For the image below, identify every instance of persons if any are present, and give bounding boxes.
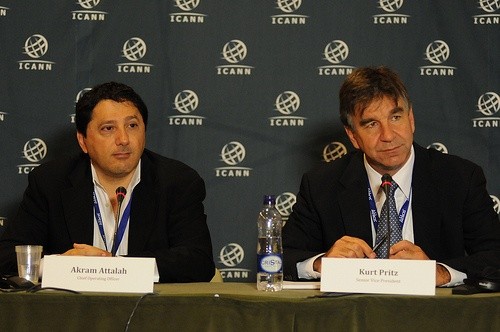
[0,81,217,285]
[279,66,499,288]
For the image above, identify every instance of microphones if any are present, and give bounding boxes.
[111,186,127,257]
[380,173,393,259]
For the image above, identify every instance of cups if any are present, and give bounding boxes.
[15,245,42,285]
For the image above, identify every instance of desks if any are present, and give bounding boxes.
[0,282,500,332]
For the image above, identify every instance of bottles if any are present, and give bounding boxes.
[255,194,285,293]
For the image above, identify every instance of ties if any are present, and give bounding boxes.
[374,176,402,259]
